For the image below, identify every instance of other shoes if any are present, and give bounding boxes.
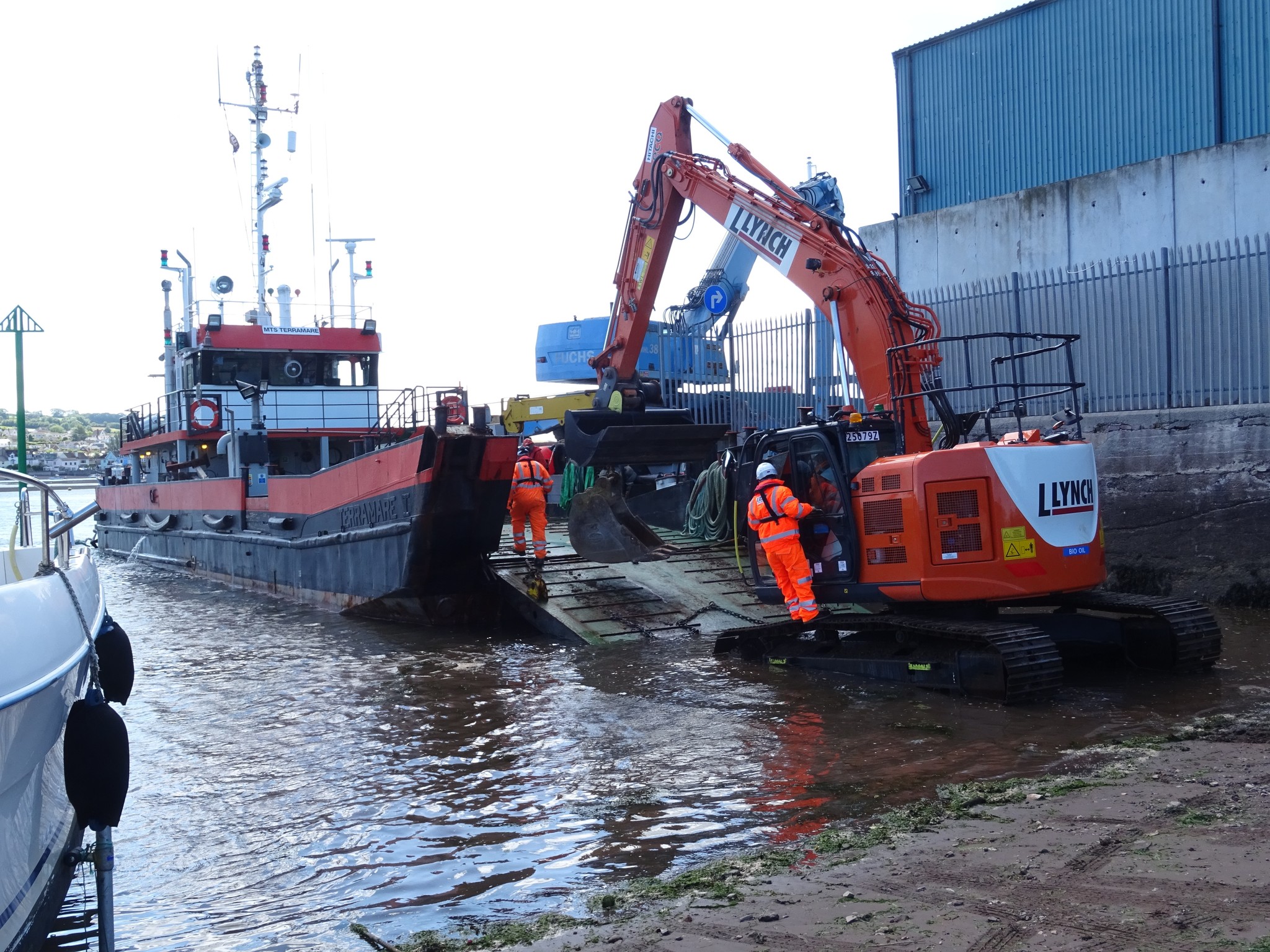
[803,610,834,624]
[513,544,526,556]
[792,618,803,623]
[534,556,544,566]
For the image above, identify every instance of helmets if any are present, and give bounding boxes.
[517,446,529,455]
[798,460,813,472]
[756,463,777,480]
[523,438,534,446]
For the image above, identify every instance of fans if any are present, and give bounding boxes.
[284,354,302,378]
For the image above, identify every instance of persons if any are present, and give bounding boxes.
[522,438,549,469]
[795,460,842,579]
[506,446,554,566]
[747,462,832,625]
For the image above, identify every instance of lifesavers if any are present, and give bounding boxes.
[441,395,466,425]
[190,399,219,430]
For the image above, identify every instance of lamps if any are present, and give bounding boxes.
[256,379,268,394]
[257,111,268,120]
[471,404,492,427]
[205,314,221,331]
[201,440,207,449]
[906,175,930,194]
[210,275,234,294]
[158,353,165,362]
[240,386,256,400]
[176,332,188,352]
[361,319,376,336]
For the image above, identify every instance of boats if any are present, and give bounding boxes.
[93,44,521,622]
[0,728,89,952]
[0,467,104,795]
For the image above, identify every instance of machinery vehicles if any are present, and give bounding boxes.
[500,94,1225,707]
